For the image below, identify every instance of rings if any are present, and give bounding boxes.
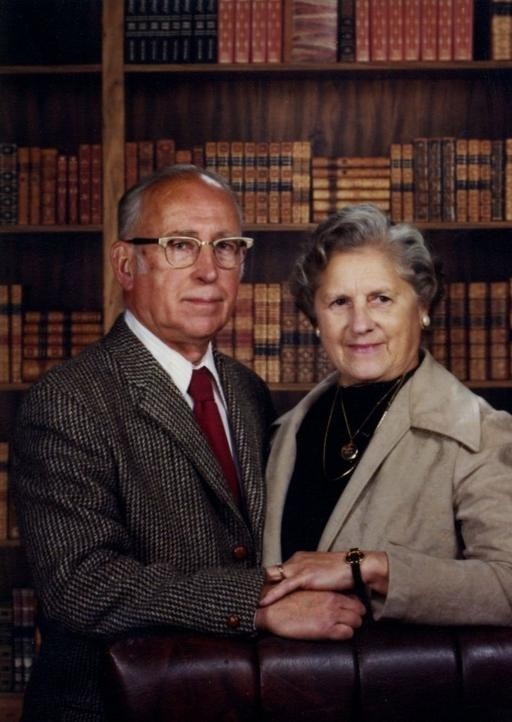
[278,564,286,581]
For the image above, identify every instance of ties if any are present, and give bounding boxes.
[187,367,242,517]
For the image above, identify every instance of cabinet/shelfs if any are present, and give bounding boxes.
[0,0,512,722]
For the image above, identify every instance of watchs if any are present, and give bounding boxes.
[344,547,365,590]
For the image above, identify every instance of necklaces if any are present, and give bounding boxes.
[319,379,407,483]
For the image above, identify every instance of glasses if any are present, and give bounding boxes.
[121,234,254,270]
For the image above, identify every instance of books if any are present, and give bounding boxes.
[126,138,511,223]
[0,3,510,65]
[423,283,511,380]
[0,284,103,383]
[1,145,105,224]
[0,443,41,692]
[213,284,336,385]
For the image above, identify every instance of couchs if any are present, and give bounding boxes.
[103,622,512,722]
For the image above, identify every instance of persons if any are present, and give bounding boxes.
[20,165,368,722]
[258,205,512,627]
